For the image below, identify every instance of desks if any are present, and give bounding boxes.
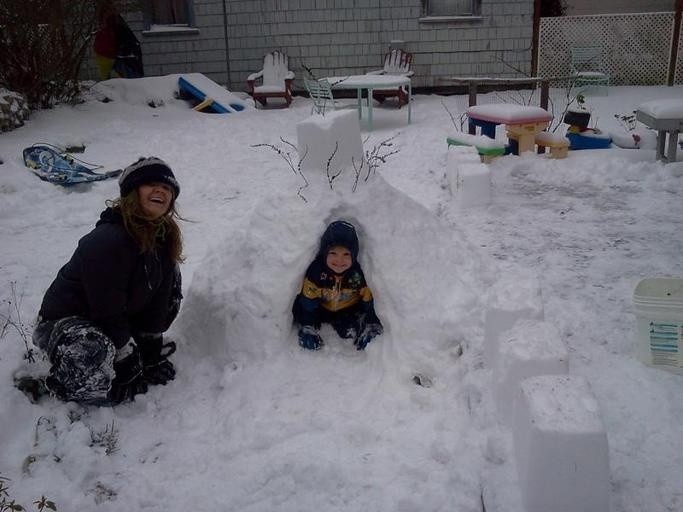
[465,106,553,156]
[318,75,412,136]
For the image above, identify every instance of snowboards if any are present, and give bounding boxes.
[23,146,123,186]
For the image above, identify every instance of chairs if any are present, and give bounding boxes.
[366,49,412,103]
[304,77,339,115]
[247,48,295,108]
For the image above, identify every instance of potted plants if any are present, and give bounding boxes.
[563,91,591,127]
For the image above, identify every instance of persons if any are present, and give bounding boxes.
[9,153,202,410]
[289,219,384,353]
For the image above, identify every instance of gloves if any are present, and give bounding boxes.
[354,323,384,351]
[297,322,323,350]
[110,348,148,406]
[137,334,175,386]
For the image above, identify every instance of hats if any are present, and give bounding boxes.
[319,220,360,266]
[119,156,180,199]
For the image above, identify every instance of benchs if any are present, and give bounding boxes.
[507,132,571,160]
[446,136,505,164]
[636,110,683,163]
